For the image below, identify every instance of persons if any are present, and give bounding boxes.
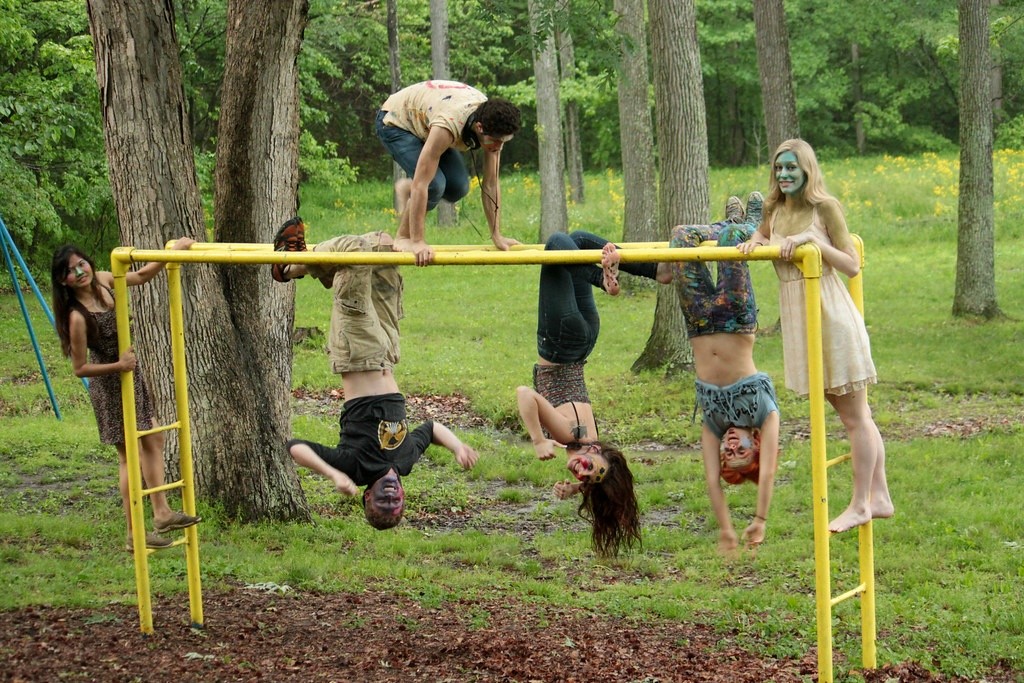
[517,231,672,560]
[375,79,521,267]
[737,140,894,533]
[271,217,481,531]
[669,192,779,559]
[52,237,202,548]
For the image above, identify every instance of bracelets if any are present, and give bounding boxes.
[755,514,766,520]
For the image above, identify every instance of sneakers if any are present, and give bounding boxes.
[271,219,298,282]
[292,216,309,279]
[726,196,746,224]
[153,510,202,534]
[746,191,764,223]
[126,530,173,551]
